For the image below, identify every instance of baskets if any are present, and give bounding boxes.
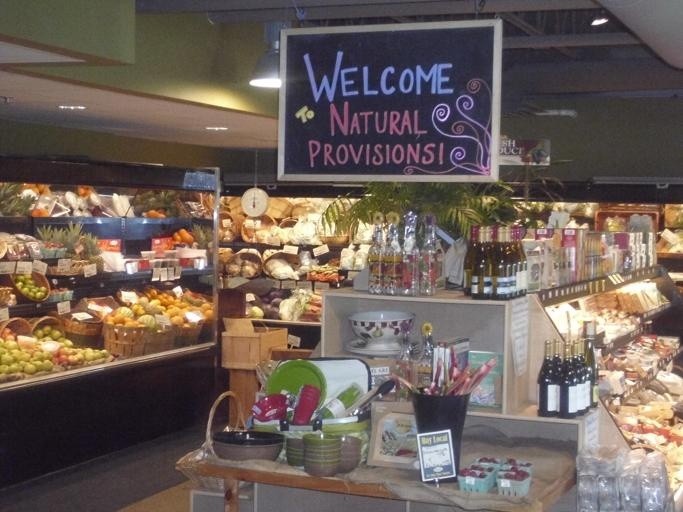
[176,194,350,290]
[175,391,252,491]
[1,257,213,358]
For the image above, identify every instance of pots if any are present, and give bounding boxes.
[286,433,362,477]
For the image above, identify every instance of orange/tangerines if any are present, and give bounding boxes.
[144,286,213,327]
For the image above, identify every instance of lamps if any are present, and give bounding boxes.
[587,8,609,27]
[249,19,292,89]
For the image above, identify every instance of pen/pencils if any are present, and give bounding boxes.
[391,342,498,398]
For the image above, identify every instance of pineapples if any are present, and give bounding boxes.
[36,222,105,275]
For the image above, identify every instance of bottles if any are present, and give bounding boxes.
[396,331,436,402]
[368,211,446,296]
[464,223,542,300]
[536,336,599,418]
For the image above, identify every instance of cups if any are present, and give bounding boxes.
[293,384,321,425]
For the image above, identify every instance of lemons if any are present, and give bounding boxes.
[106,315,145,330]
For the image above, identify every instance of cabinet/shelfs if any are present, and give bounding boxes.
[213,195,682,323]
[0,156,215,386]
[534,264,683,510]
[188,289,603,508]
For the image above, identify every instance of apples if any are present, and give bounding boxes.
[0,274,110,381]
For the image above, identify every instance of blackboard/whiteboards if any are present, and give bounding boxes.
[277,19,504,183]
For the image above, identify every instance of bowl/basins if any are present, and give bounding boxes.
[348,310,415,350]
[256,394,287,422]
[213,431,284,461]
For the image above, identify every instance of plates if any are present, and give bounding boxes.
[265,360,327,418]
[346,339,401,356]
